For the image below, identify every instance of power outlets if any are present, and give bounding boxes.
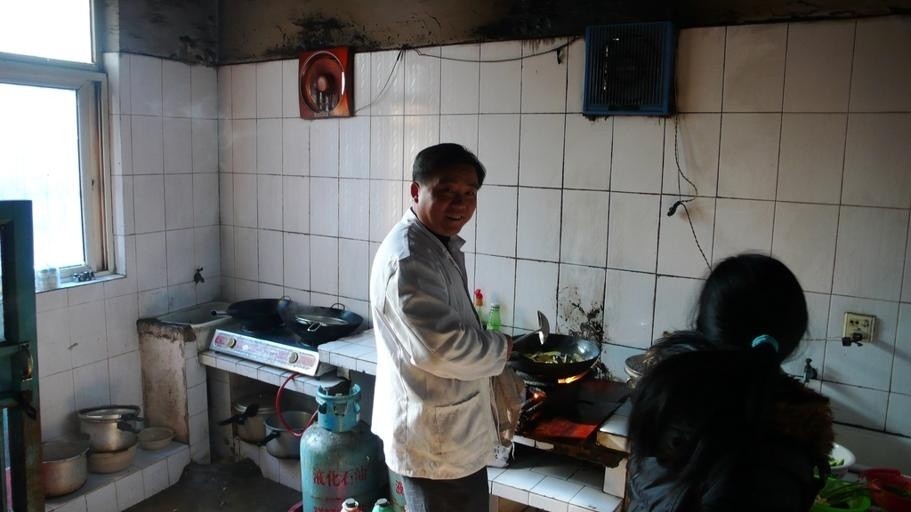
[842,311,876,344]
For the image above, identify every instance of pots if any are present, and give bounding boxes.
[41,440,89,499]
[504,333,601,379]
[76,401,144,453]
[219,390,289,444]
[257,411,316,459]
[211,293,294,329]
[284,301,364,344]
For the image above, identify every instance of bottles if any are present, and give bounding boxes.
[40,266,58,290]
[473,288,484,331]
[486,302,502,331]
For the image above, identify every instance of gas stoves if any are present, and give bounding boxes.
[208,324,335,378]
[514,369,594,433]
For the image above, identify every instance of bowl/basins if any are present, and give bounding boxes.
[828,442,857,478]
[89,440,140,475]
[137,426,175,451]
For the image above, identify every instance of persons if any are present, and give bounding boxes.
[623,254,834,511]
[370,143,512,512]
